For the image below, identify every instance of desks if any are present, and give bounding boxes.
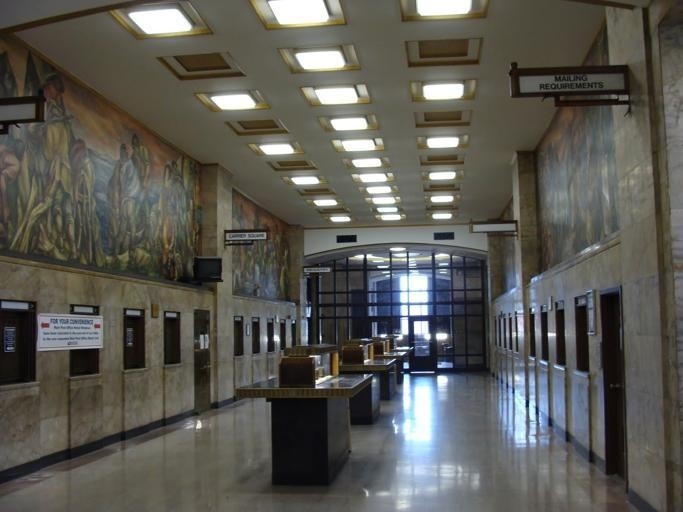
[235,347,415,485]
